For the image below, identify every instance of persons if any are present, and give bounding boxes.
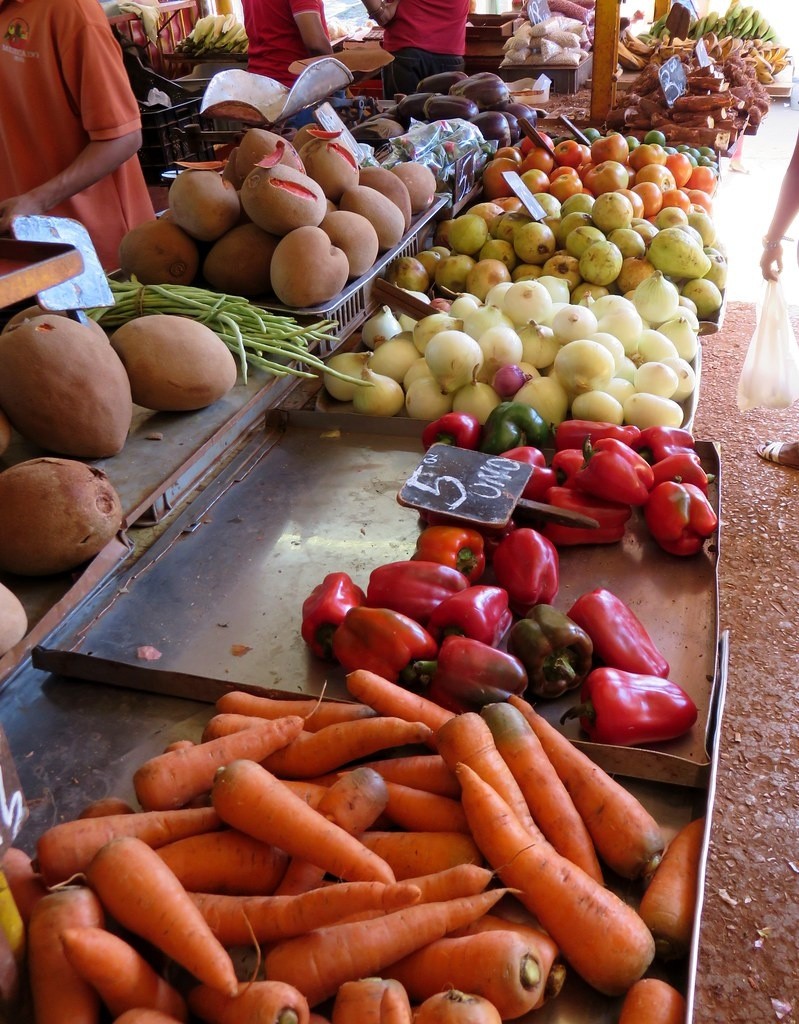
[239,0,334,91]
[755,129,799,470]
[1,0,161,277]
[361,0,477,101]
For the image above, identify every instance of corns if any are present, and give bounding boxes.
[175,13,249,54]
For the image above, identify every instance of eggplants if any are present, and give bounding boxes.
[347,71,537,147]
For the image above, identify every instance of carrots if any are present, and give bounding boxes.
[1,667,705,1024]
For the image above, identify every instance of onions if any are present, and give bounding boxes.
[322,277,699,432]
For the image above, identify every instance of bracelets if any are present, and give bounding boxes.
[367,2,386,20]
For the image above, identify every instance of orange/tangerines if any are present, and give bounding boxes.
[553,127,718,170]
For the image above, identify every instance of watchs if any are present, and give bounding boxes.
[762,235,781,251]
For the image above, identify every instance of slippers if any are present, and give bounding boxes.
[758,441,799,471]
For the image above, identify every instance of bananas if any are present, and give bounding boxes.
[615,0,791,84]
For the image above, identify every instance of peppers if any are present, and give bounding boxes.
[298,402,717,745]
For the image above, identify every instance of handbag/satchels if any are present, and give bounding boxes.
[737,270,799,412]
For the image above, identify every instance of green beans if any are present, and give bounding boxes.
[83,274,373,387]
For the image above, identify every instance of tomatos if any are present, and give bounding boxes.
[468,132,717,225]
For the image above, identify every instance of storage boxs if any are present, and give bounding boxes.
[497,51,594,96]
[137,96,219,188]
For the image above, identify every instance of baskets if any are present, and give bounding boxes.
[242,196,451,359]
[499,51,593,94]
